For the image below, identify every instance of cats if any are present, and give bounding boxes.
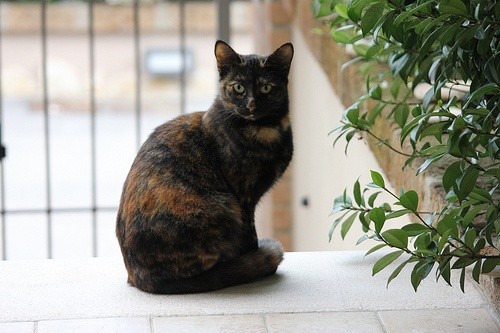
[115,40,294,295]
